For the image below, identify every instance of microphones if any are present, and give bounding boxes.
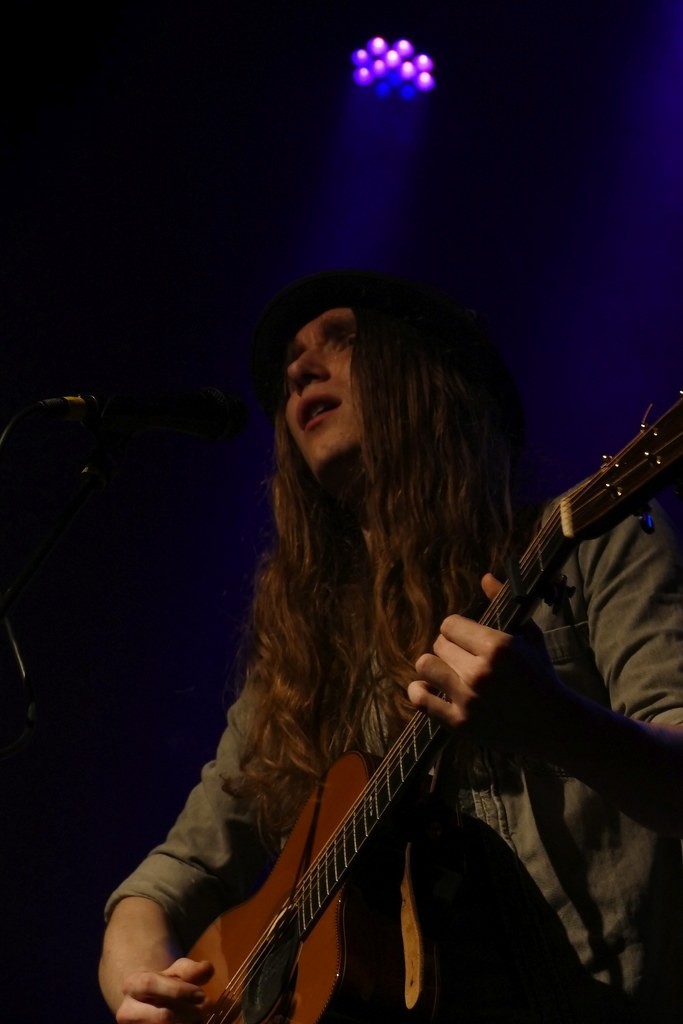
[39,386,250,442]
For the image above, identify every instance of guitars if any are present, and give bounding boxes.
[177,380,673,1023]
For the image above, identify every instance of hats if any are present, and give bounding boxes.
[252,270,527,450]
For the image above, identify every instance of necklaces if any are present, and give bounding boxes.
[362,571,387,750]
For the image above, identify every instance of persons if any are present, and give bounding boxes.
[99,269,683,1024]
